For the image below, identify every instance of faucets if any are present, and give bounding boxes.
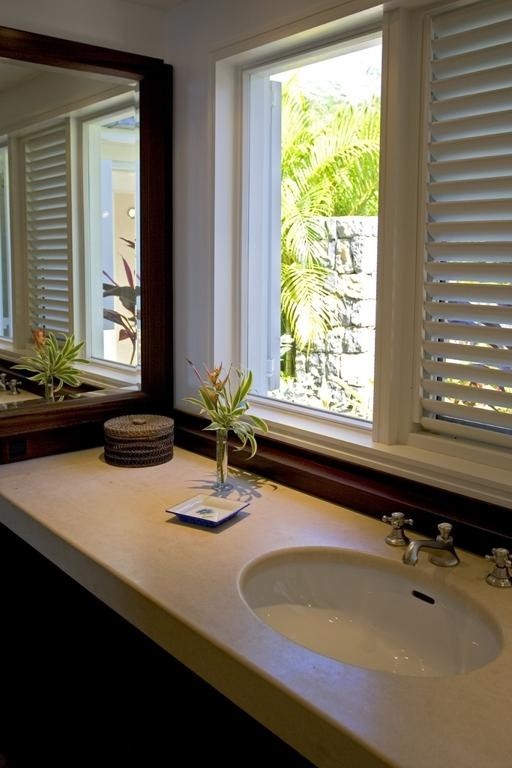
[401,521,461,568]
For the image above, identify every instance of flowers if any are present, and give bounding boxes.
[10,326,90,403]
[181,357,268,461]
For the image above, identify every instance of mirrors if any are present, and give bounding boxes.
[0,26,173,464]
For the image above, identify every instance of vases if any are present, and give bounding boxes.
[45,377,54,402]
[212,428,233,492]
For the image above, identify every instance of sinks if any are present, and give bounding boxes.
[235,545,501,677]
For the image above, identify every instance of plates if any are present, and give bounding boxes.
[166,494,250,528]
